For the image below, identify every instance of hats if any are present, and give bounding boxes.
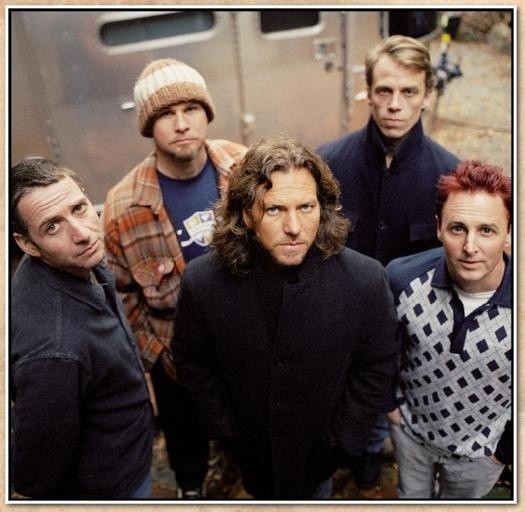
[132,59,214,138]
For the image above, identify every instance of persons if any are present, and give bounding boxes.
[383,156,515,501]
[9,154,156,498]
[314,33,473,502]
[101,55,253,500]
[167,132,406,501]
